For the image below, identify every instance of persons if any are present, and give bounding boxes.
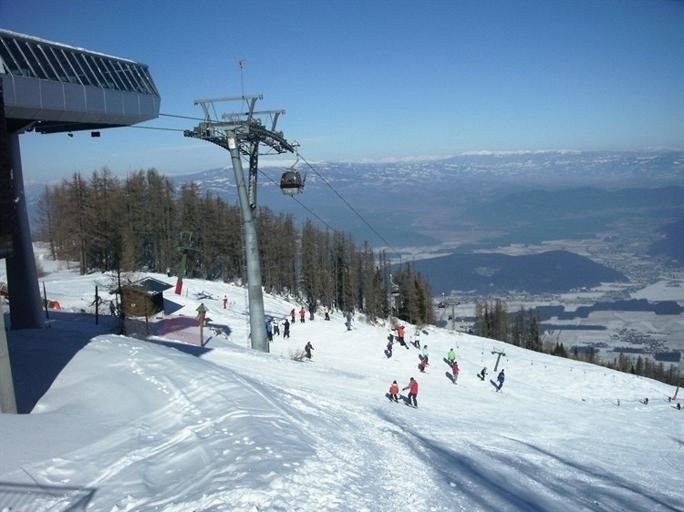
[481,367,487,380]
[345,310,353,330]
[403,377,418,408]
[305,342,314,359]
[265,302,330,341]
[447,349,455,365]
[389,380,399,401]
[452,361,459,384]
[110,301,116,316]
[385,325,429,372]
[497,369,504,389]
[222,295,228,309]
